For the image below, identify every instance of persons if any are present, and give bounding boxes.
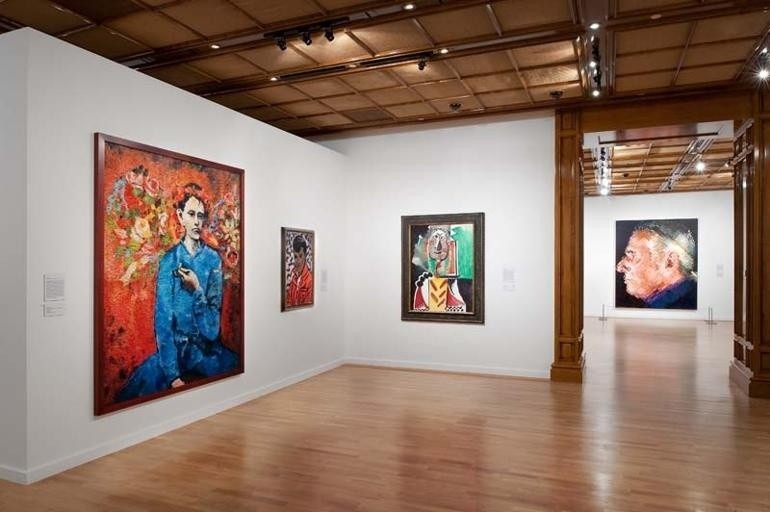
[286,235,313,306]
[115,182,239,403]
[616,220,698,310]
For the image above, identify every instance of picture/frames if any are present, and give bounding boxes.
[88,129,248,421]
[398,211,487,326]
[277,223,317,315]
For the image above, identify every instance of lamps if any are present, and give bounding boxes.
[416,54,427,71]
[590,31,605,96]
[257,11,353,54]
